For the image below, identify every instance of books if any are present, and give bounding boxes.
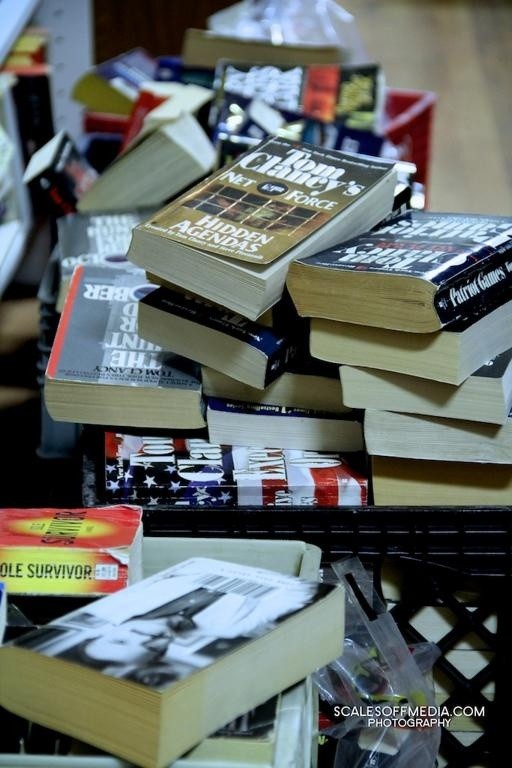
[205,59,387,157]
[136,286,310,391]
[72,46,162,116]
[287,212,512,333]
[200,356,353,413]
[77,115,219,214]
[125,138,399,322]
[339,353,512,427]
[0,702,282,768]
[23,129,98,216]
[307,300,512,385]
[370,452,512,509]
[42,266,207,428]
[205,394,363,451]
[52,208,158,314]
[105,431,370,512]
[0,506,146,597]
[363,405,512,466]
